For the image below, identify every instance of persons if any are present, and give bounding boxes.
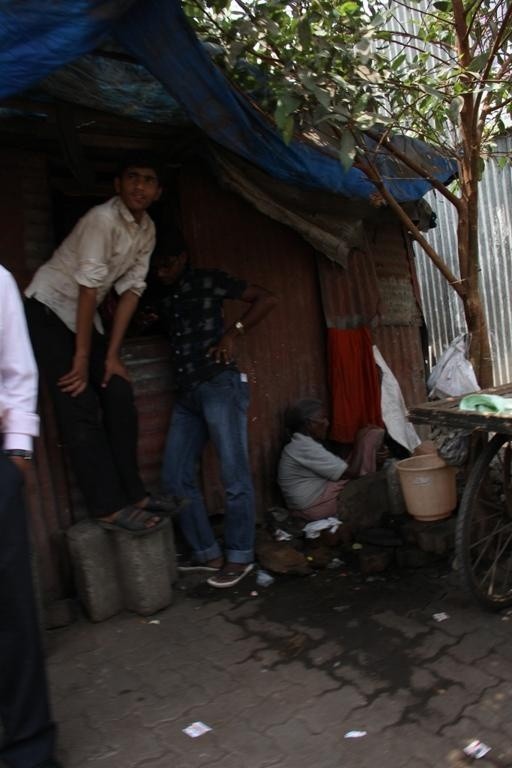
[22,150,170,535]
[1,263,63,768]
[276,397,391,528]
[130,226,282,589]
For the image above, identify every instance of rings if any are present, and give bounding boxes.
[222,348,227,352]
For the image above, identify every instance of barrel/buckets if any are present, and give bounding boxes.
[394,454,461,523]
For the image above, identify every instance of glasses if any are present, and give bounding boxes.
[153,258,180,271]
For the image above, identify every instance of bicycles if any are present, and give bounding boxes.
[410,380,512,614]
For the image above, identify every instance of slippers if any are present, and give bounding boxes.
[207,560,258,588]
[137,493,187,516]
[175,557,225,573]
[100,507,170,536]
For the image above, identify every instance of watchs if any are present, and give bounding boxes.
[4,449,32,462]
[230,320,245,335]
